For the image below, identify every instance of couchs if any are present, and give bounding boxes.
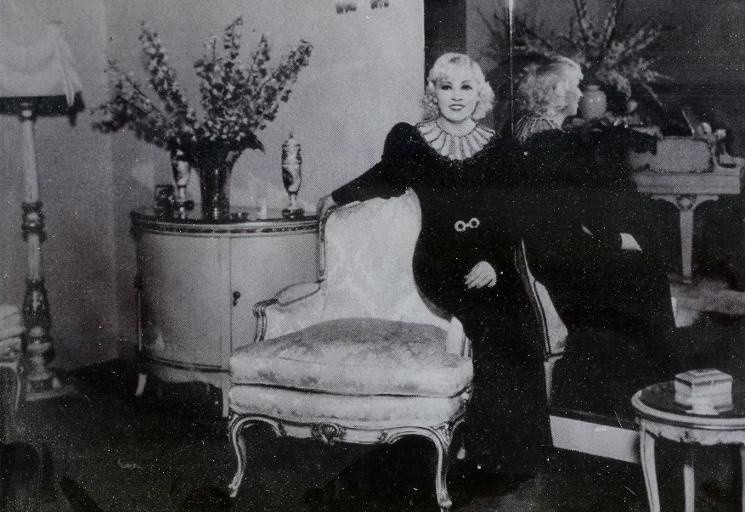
[228,190,475,508]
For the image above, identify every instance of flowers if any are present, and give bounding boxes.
[91,18,315,165]
[507,0,678,114]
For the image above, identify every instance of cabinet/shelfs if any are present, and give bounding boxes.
[130,205,320,418]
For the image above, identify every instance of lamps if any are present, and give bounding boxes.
[0,20,84,401]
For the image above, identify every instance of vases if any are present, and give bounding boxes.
[200,153,232,224]
[578,84,609,122]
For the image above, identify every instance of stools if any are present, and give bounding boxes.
[632,381,744,512]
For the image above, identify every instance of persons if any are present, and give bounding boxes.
[317,51,554,496]
[500,54,679,423]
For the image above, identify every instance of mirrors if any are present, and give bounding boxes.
[423,1,744,431]
